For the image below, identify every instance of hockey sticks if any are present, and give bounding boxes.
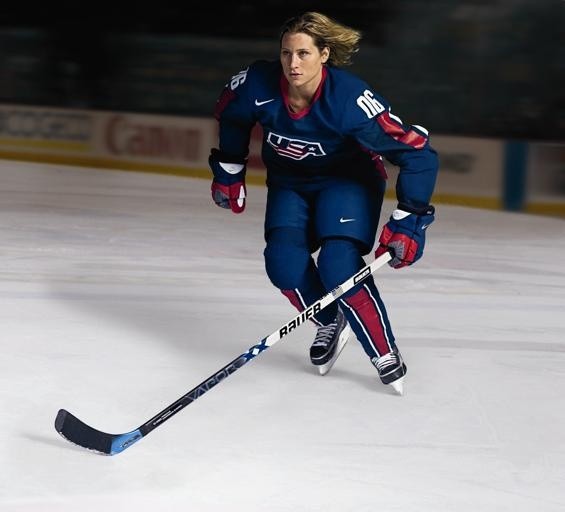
[55,205,437,454]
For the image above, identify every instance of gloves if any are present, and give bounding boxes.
[208,147,246,212]
[373,203,434,270]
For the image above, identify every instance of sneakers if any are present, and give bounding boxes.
[370,345,408,384]
[310,312,344,366]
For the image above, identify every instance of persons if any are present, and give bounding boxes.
[208,11,439,385]
